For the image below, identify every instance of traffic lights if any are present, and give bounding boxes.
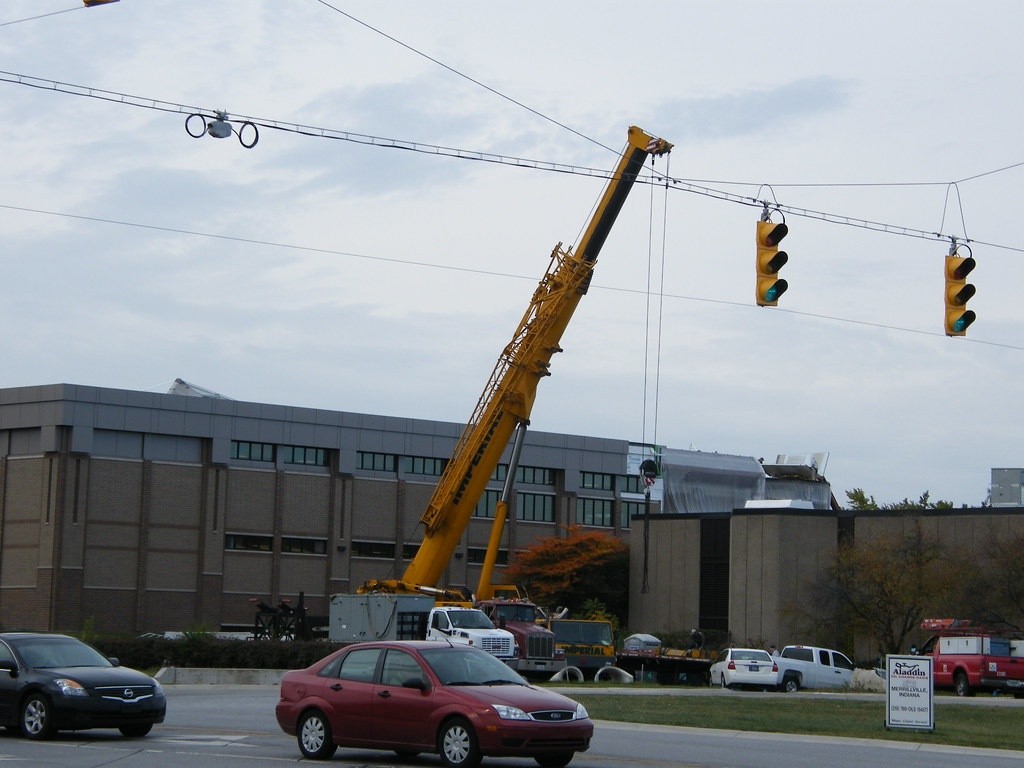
[756,219,789,308]
[943,255,978,335]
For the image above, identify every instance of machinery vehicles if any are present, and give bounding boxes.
[355,125,676,667]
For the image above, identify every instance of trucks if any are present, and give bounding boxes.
[329,593,568,679]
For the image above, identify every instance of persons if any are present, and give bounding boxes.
[770,645,780,657]
[691,629,705,649]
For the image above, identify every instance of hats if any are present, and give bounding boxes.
[691,629,696,635]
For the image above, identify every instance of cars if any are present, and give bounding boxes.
[709,648,778,691]
[0,632,167,739]
[276,640,594,768]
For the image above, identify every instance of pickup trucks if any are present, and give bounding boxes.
[771,645,860,693]
[909,618,1024,699]
